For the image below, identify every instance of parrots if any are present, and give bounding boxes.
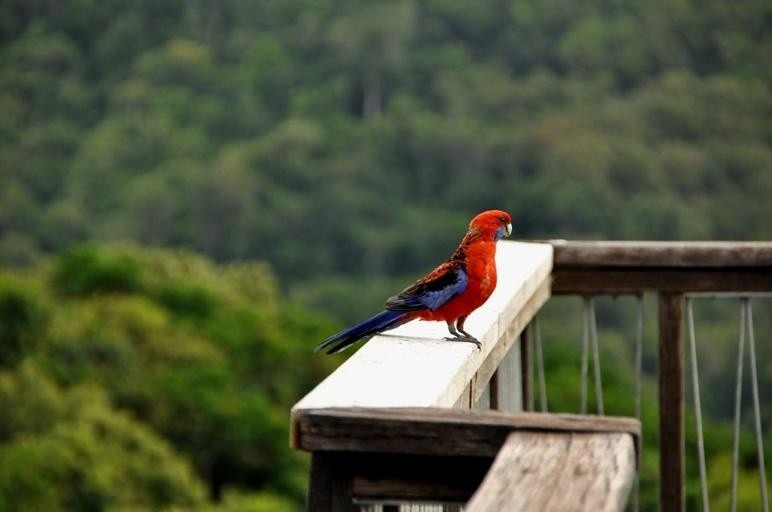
[314,208,514,356]
